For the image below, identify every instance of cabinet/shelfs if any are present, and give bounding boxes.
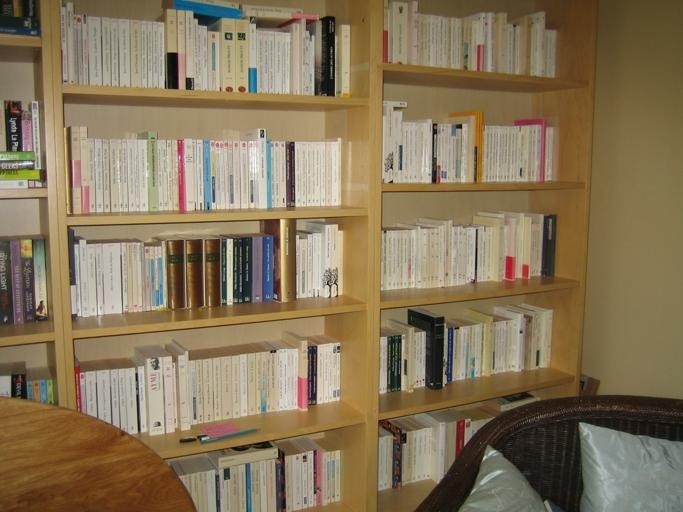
[367,0,601,512]
[53,1,374,512]
[0,1,68,408]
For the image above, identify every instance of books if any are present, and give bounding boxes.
[60,0,352,99]
[1,1,40,37]
[73,331,340,435]
[65,124,341,215]
[0,235,50,326]
[380,302,553,396]
[1,361,56,407]
[382,99,559,186]
[382,209,556,289]
[377,393,542,491]
[2,100,45,188]
[382,0,557,77]
[68,219,345,318]
[166,438,341,510]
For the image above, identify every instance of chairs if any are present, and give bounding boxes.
[413,394,683,512]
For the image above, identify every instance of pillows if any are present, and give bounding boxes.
[459,443,552,511]
[577,420,683,512]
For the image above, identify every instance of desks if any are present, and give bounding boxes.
[0,395,199,512]
